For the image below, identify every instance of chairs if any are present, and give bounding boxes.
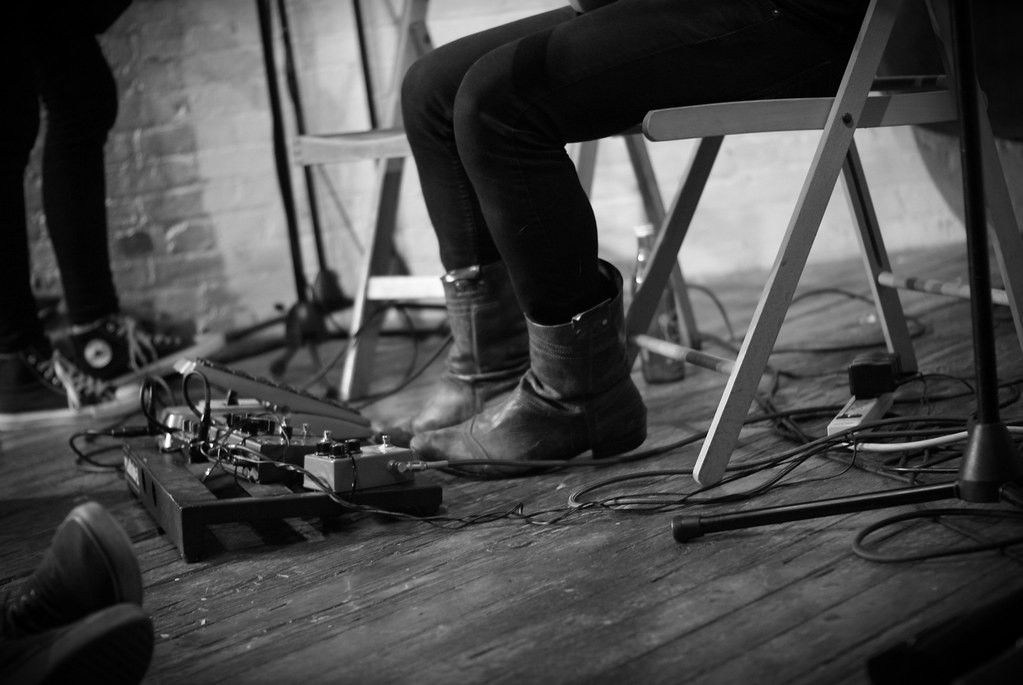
[296,0,1023,489]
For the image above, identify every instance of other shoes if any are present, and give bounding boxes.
[13,602,155,685]
[0,502,144,630]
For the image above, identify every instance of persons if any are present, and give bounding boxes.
[0,0,228,436]
[359,0,1023,480]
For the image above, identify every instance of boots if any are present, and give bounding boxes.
[370,263,528,446]
[412,258,648,480]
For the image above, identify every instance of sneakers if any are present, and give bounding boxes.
[68,316,225,386]
[0,339,150,431]
